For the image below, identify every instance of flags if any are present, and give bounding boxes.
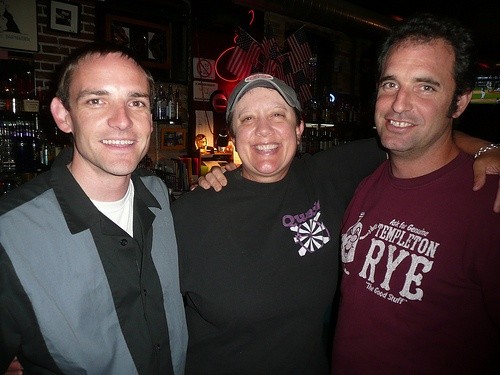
[222,16,318,105]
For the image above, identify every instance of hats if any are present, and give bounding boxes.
[226,71,302,118]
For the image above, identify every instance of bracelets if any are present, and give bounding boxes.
[473,143,499,160]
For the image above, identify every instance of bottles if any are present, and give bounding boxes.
[0,85,360,197]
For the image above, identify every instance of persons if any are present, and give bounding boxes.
[190,15,500,375]
[4,72,500,375]
[0,49,197,374]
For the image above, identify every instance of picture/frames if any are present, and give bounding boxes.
[0,0,39,53]
[47,1,82,36]
[160,128,186,150]
